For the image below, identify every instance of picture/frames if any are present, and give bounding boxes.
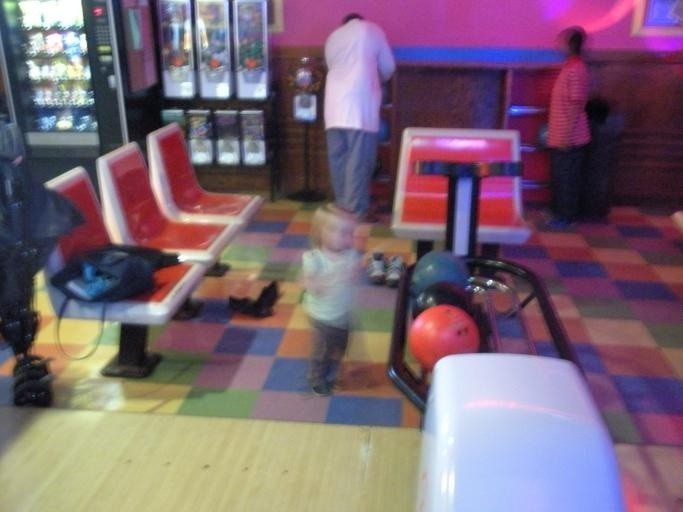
[629,1,683,38]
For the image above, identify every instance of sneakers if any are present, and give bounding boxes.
[310,381,329,397]
[366,251,386,282]
[386,255,403,286]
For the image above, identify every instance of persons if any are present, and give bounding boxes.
[322,14,396,220]
[547,27,592,232]
[298,204,368,397]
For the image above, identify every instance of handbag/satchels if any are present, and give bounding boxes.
[52,249,153,361]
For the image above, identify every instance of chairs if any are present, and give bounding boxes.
[146,123,262,276]
[39,164,206,379]
[390,127,531,288]
[94,139,240,322]
